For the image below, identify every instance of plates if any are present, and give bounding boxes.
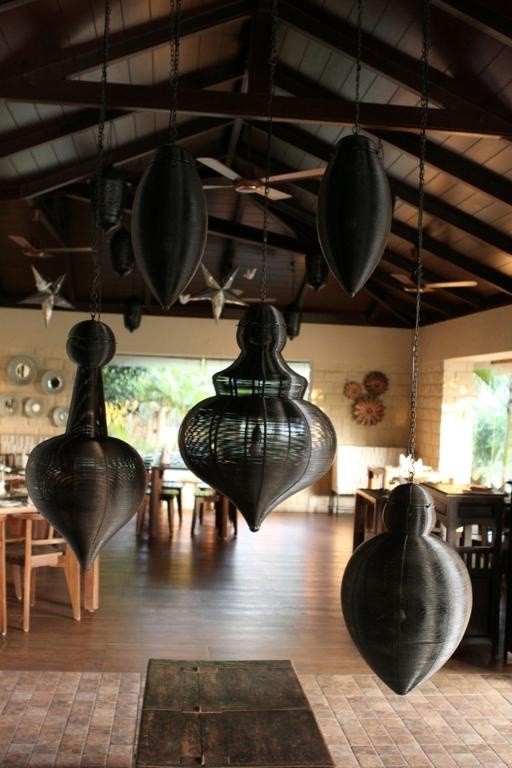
[0,354,69,427]
[470,486,493,491]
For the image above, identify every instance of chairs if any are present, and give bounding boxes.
[6,513,102,633]
